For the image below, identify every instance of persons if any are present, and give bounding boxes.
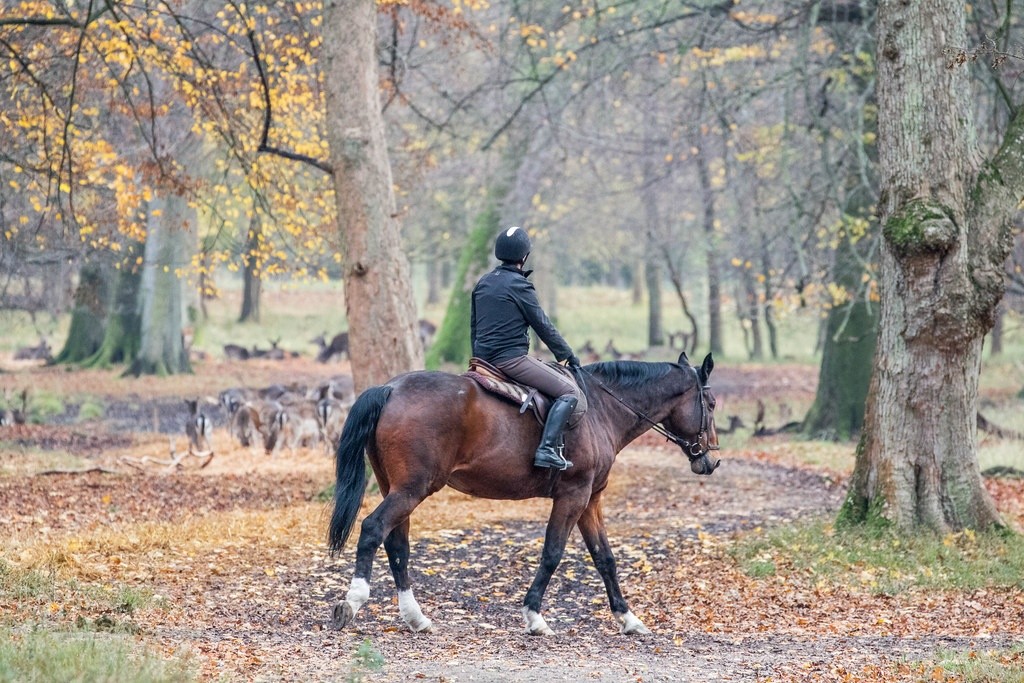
[471,225,581,468]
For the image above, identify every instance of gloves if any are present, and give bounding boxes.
[567,355,580,368]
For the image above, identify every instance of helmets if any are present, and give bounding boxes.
[494,227,530,262]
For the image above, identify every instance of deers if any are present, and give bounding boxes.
[182,321,766,457]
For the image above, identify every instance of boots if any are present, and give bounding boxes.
[534,395,573,468]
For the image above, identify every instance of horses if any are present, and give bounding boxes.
[322,346,727,635]
